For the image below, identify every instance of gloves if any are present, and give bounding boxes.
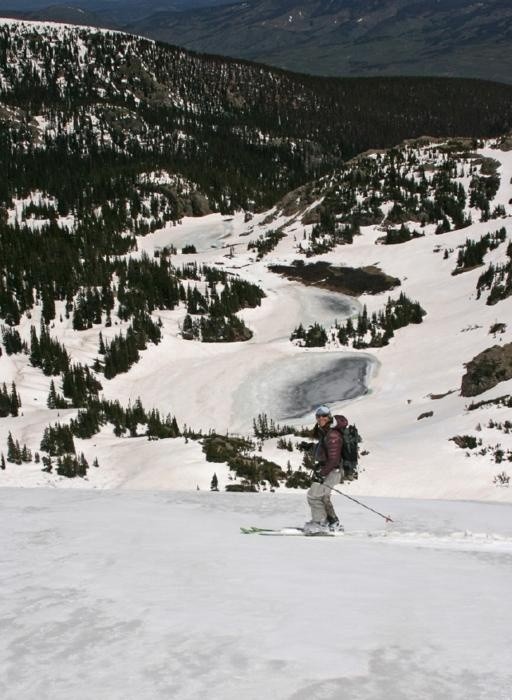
[313,470,324,485]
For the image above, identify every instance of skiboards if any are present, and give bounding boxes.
[240,527,388,539]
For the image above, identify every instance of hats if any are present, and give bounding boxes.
[316,406,331,418]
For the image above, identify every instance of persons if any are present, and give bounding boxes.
[303,405,343,533]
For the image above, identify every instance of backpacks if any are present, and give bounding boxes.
[323,423,358,481]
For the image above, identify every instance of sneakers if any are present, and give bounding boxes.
[328,520,340,529]
[305,519,326,529]
